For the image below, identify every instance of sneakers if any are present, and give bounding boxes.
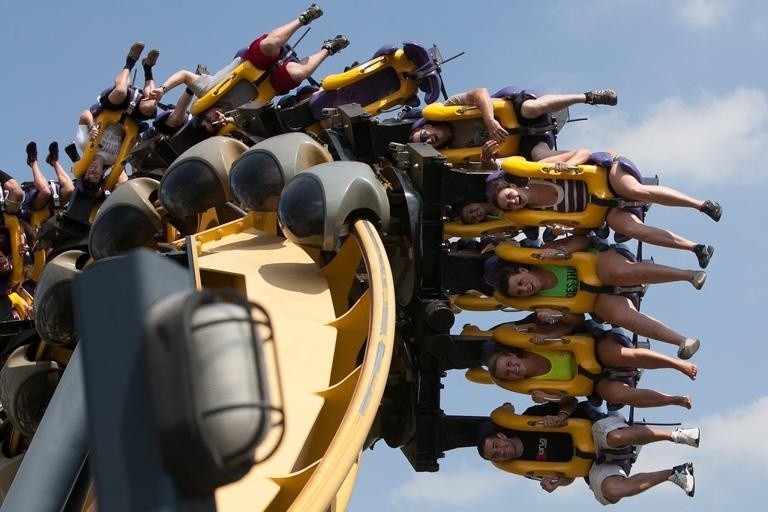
[677,337,700,360]
[195,63,209,76]
[127,41,145,62]
[693,244,714,268]
[45,141,59,166]
[583,88,618,105]
[667,460,696,497]
[690,270,707,290]
[698,198,723,222]
[321,34,351,57]
[671,426,701,448]
[141,49,160,68]
[25,141,38,167]
[298,3,323,26]
[64,141,80,163]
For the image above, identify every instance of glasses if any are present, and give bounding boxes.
[419,128,430,143]
